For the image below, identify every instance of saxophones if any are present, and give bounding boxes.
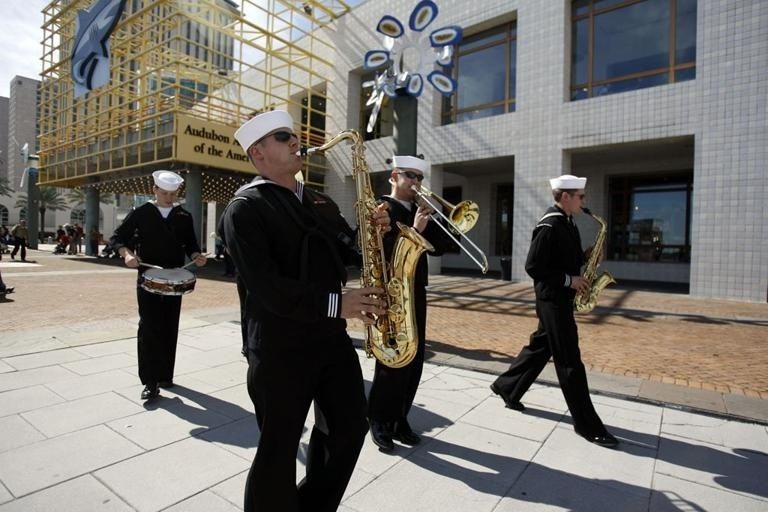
[295,128,435,369]
[574,206,616,315]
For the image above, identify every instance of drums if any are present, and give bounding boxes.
[140,267,197,296]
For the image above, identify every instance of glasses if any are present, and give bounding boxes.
[396,171,424,181]
[254,131,297,146]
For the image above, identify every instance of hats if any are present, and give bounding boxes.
[152,170,184,191]
[549,175,587,189]
[234,110,293,151]
[393,155,425,172]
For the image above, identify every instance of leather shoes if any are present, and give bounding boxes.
[490,382,525,411]
[141,382,160,399]
[576,430,619,447]
[393,432,420,445]
[367,417,395,449]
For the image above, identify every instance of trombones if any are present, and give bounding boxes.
[389,173,490,273]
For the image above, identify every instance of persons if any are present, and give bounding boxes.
[110,170,207,399]
[215,109,388,512]
[489,174,620,447]
[54,223,83,254]
[366,156,447,450]
[103,226,140,259]
[0,220,29,303]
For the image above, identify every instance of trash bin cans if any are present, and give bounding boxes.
[80,236,84,244]
[47,235,53,245]
[498,257,512,280]
